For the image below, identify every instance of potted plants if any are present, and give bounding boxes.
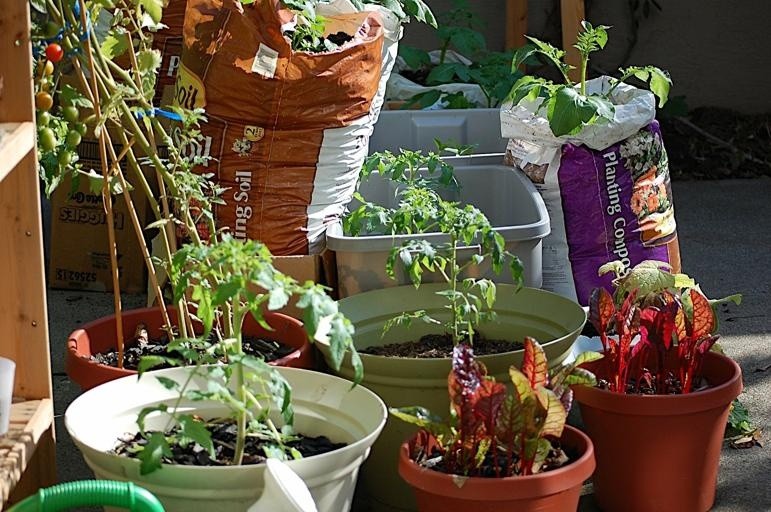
[569,260,744,512]
[65,235,387,512]
[326,165,550,300]
[368,28,541,168]
[29,0,308,394]
[314,187,588,512]
[388,336,604,512]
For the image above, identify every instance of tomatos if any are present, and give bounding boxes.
[32,42,87,166]
